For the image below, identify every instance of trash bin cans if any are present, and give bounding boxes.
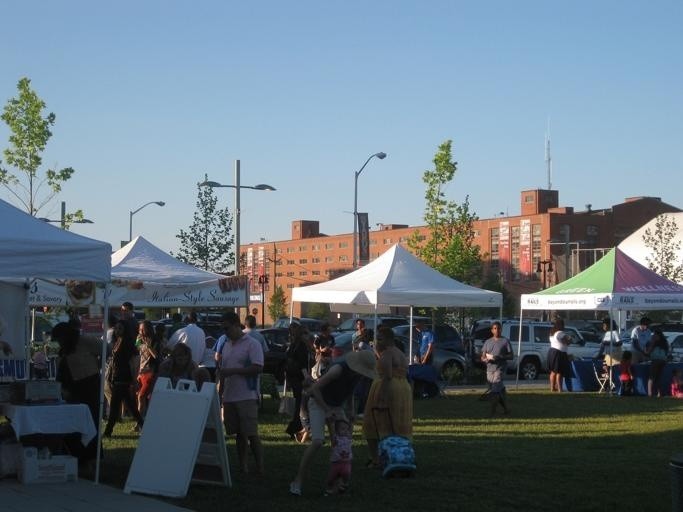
[670,453,683,512]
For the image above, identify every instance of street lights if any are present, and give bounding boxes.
[257,274,269,328]
[353,151,388,270]
[199,158,278,321]
[127,201,166,242]
[30,202,94,232]
[535,260,553,322]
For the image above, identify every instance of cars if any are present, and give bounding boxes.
[255,328,294,366]
[29,311,72,344]
[326,316,468,384]
[77,310,246,362]
[574,323,682,364]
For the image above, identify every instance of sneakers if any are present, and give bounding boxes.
[289,480,301,495]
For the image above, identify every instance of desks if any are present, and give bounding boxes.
[0,399,99,460]
[605,359,682,399]
[407,362,437,397]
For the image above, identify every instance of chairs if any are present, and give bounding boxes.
[436,361,459,398]
[591,358,616,394]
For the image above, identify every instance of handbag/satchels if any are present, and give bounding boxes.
[278,397,295,417]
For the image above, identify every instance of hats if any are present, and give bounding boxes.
[345,350,377,381]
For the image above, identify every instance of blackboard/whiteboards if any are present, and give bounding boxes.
[125,377,216,499]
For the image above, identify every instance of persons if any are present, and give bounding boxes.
[51,301,413,499]
[548,319,572,392]
[630,317,652,363]
[593,319,622,395]
[619,351,634,396]
[481,322,514,418]
[670,369,683,399]
[415,322,441,399]
[644,329,669,398]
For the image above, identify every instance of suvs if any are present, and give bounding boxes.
[270,316,330,344]
[468,319,604,380]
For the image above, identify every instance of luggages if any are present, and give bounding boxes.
[370,407,417,479]
